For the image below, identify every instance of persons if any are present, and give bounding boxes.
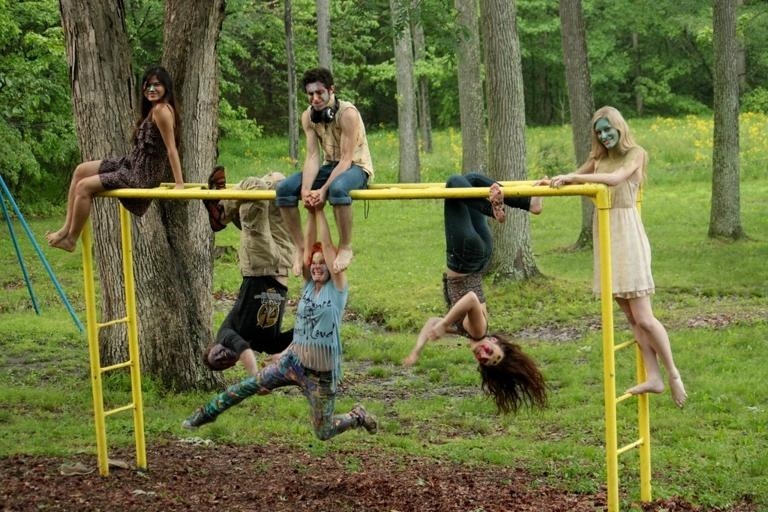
[551,106,688,409]
[182,192,378,441]
[404,171,550,418]
[44,67,183,251]
[200,166,295,395]
[275,67,374,277]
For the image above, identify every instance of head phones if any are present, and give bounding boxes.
[311,106,335,124]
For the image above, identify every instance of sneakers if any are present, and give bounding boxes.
[183,409,217,429]
[202,168,227,231]
[353,404,377,436]
